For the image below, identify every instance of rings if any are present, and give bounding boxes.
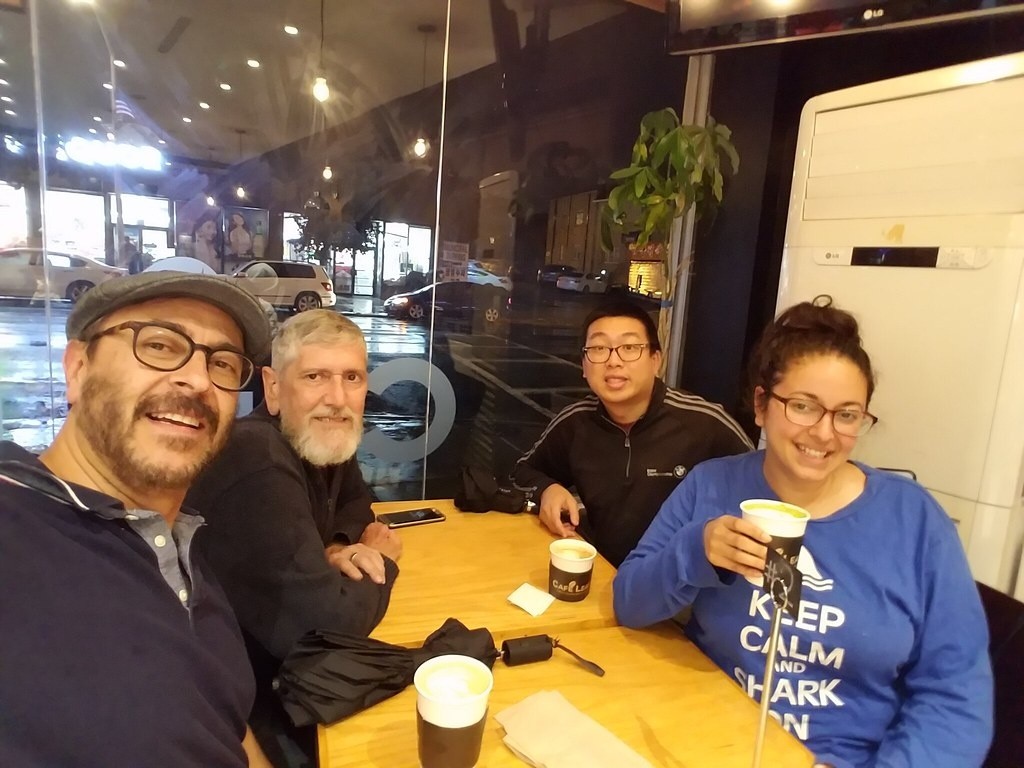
[350,552,358,562]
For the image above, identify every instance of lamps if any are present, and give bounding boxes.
[236,185,245,197]
[323,162,333,178]
[313,65,329,102]
[415,130,428,159]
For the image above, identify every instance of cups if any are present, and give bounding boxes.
[414,654,493,768]
[739,498,811,588]
[548,538,597,603]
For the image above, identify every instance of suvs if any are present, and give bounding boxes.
[217,259,337,317]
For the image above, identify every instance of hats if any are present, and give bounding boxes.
[66,256,270,369]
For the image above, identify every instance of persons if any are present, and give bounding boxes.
[227,212,252,257]
[124,236,136,260]
[130,252,144,276]
[0,268,277,768]
[179,310,403,767]
[610,295,998,768]
[191,212,221,273]
[494,301,754,571]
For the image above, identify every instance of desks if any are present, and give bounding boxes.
[318,499,826,768]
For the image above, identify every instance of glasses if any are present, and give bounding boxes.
[768,391,878,437]
[88,322,259,391]
[582,344,650,364]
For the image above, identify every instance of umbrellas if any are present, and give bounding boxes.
[272,616,604,729]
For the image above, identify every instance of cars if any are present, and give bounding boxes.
[536,264,573,285]
[467,261,514,293]
[382,282,513,322]
[0,248,130,304]
[556,269,612,296]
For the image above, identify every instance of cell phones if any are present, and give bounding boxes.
[376,508,446,529]
